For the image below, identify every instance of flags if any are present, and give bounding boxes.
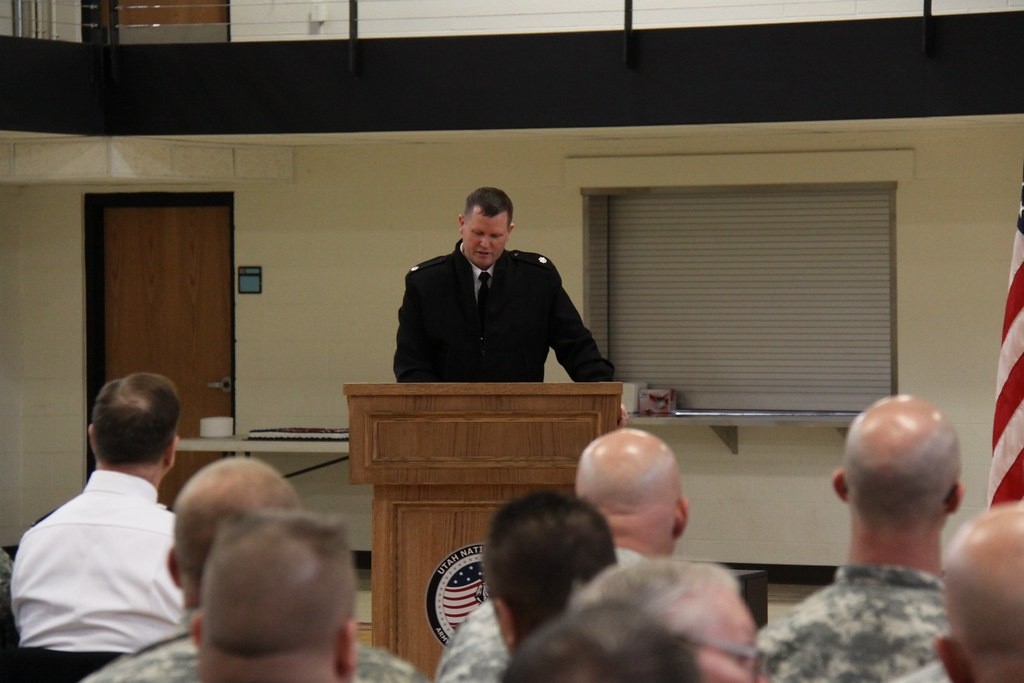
[987,169,1024,507]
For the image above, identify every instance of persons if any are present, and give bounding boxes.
[498,596,705,683]
[434,428,688,683]
[935,501,1024,683]
[482,491,620,663]
[78,456,428,683]
[192,503,359,683]
[392,186,629,430]
[10,372,190,655]
[567,556,770,683]
[755,394,966,683]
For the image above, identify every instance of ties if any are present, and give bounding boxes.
[478,272,491,310]
